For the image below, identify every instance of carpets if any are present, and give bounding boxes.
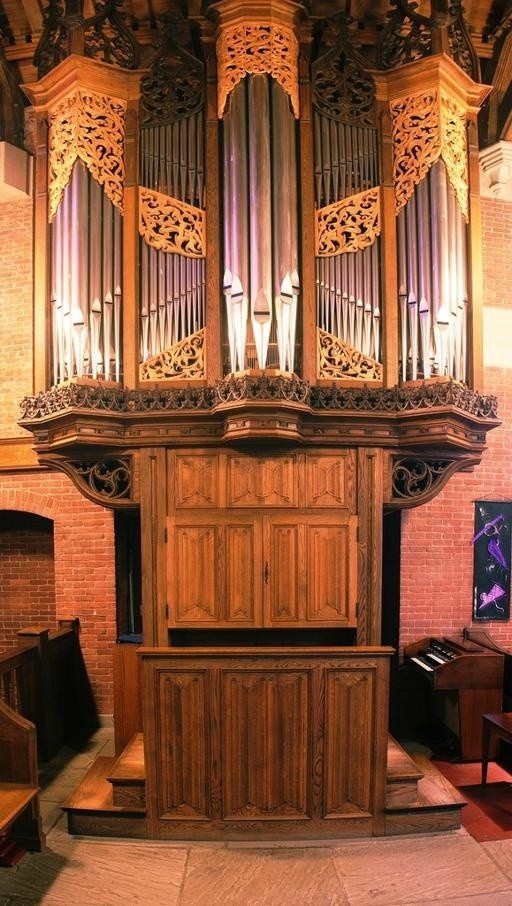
[449,780,511,842]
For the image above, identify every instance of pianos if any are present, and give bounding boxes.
[402,636,505,761]
